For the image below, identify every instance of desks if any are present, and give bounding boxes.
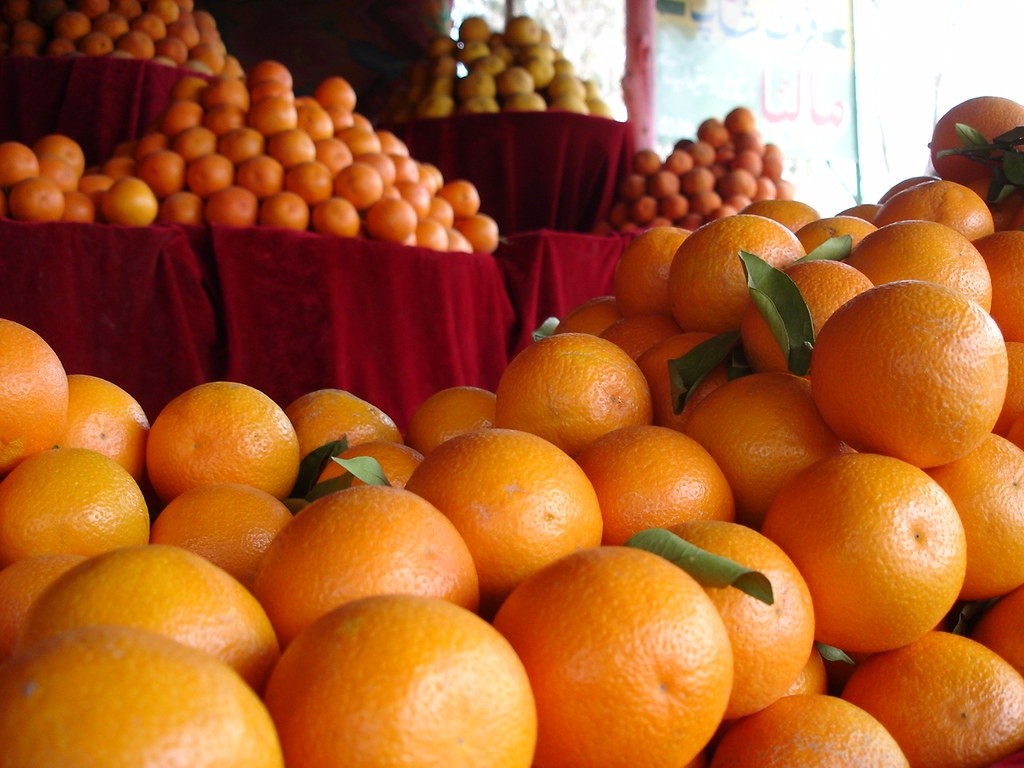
[0,0,651,408]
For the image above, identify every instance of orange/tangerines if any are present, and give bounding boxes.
[1,94,1024,768]
[0,0,500,256]
[380,15,609,119]
